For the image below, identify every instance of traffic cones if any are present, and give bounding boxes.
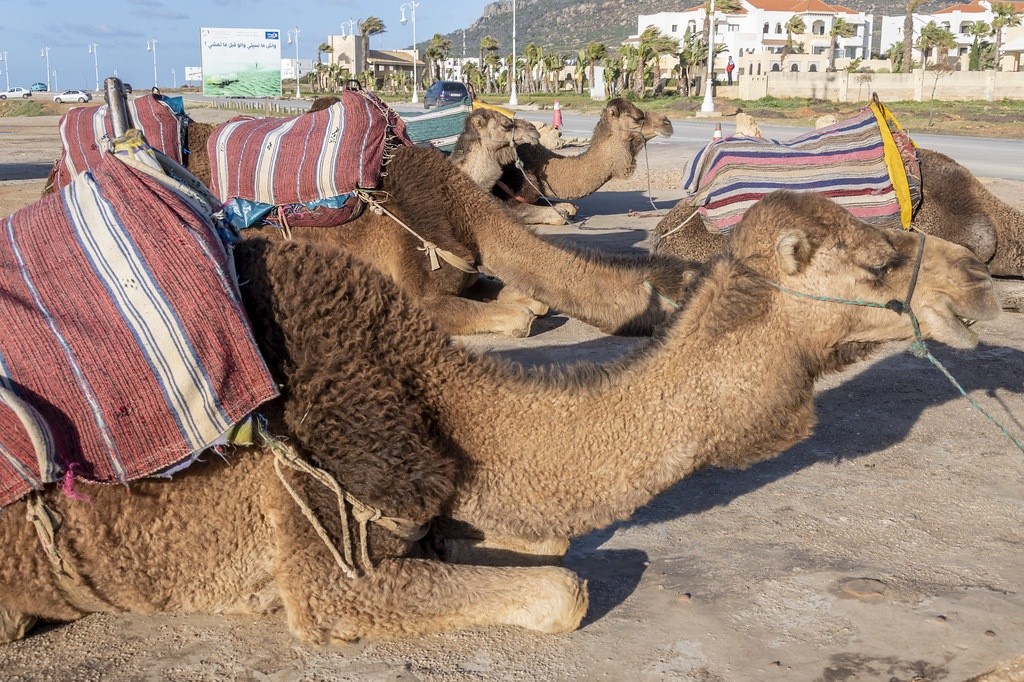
[551,100,563,128]
[713,123,722,141]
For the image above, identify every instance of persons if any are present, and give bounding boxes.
[726,55,735,85]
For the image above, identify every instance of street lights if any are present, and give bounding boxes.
[88,42,100,92]
[341,17,357,87]
[41,45,51,91]
[114,69,117,78]
[53,70,57,92]
[400,0,419,103]
[147,38,158,88]
[172,68,176,89]
[287,27,302,99]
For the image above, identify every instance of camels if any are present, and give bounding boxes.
[0,73,1024,645]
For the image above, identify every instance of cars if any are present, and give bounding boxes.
[31,83,47,92]
[54,90,92,103]
[0,87,32,100]
[123,83,132,94]
[0,49,9,93]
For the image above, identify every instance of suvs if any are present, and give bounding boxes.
[424,81,468,109]
